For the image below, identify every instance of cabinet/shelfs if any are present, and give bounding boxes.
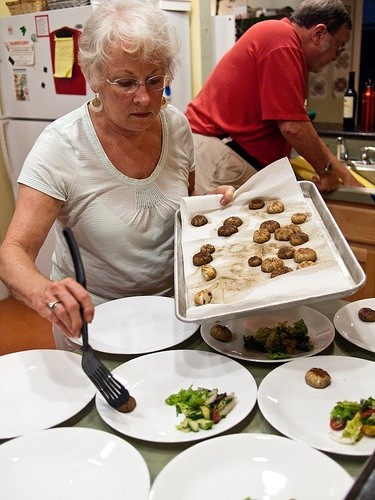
[326,200,375,302]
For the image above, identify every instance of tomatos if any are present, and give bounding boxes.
[330,417,346,430]
[210,409,219,424]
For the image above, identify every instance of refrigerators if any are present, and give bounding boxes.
[1,6,193,284]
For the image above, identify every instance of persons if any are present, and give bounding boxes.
[0,1,236,349]
[184,0,356,193]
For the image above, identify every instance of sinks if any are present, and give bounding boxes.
[331,162,375,192]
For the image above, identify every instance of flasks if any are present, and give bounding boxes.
[357,77,375,133]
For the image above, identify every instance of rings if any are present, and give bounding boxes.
[48,300,61,311]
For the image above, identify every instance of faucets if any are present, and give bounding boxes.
[336,136,349,160]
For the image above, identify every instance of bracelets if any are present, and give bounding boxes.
[320,159,337,175]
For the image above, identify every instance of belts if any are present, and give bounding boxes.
[223,137,264,172]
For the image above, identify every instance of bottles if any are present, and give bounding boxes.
[336,137,348,162]
[342,70,357,131]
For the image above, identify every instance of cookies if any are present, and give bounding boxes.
[210,323,232,342]
[189,199,318,305]
[305,368,332,389]
[116,396,136,413]
[357,308,375,322]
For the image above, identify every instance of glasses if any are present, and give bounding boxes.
[102,67,175,94]
[332,34,346,57]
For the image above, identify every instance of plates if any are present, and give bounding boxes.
[0,427,151,500]
[95,348,258,444]
[0,348,98,441]
[257,355,375,456]
[198,304,336,363]
[148,431,358,500]
[68,295,202,354]
[332,297,375,355]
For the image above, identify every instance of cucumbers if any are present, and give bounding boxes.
[197,418,213,430]
[188,421,199,432]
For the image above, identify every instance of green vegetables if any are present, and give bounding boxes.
[330,396,375,444]
[241,318,313,360]
[165,384,227,432]
